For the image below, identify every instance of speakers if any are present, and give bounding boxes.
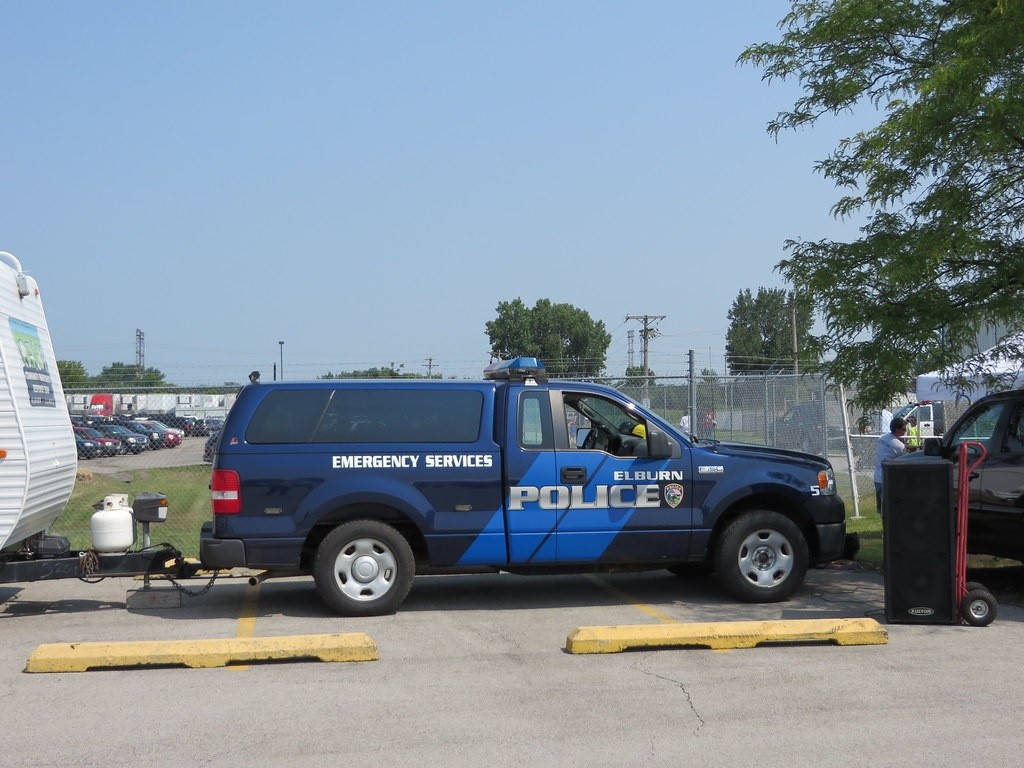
[883,450,954,625]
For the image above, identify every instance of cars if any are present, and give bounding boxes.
[75,433,100,460]
[70,414,133,426]
[150,414,226,437]
[895,388,1024,564]
[145,420,185,448]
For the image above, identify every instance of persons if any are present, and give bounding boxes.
[679,408,717,439]
[879,406,894,434]
[568,413,579,447]
[874,416,918,519]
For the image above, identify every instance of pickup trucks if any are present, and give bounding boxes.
[201,370,860,618]
[765,400,857,456]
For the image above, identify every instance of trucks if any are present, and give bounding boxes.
[896,400,996,439]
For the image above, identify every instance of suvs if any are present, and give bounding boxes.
[73,427,121,457]
[121,421,166,450]
[99,425,150,455]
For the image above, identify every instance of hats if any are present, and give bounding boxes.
[910,417,917,425]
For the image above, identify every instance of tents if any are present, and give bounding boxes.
[916,331,1024,453]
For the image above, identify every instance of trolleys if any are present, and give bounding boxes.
[954,441,998,627]
